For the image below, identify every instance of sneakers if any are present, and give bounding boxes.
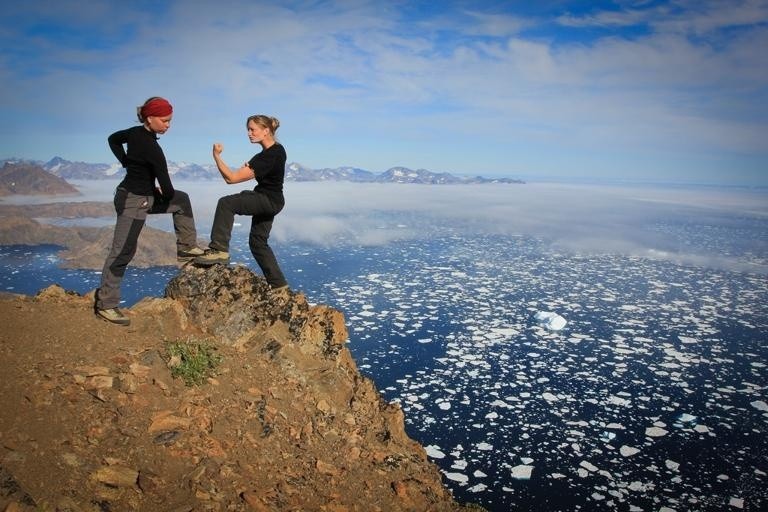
[177,244,231,266]
[96,307,130,326]
[272,284,289,292]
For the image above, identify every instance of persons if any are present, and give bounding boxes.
[95,97,204,326]
[194,115,289,292]
